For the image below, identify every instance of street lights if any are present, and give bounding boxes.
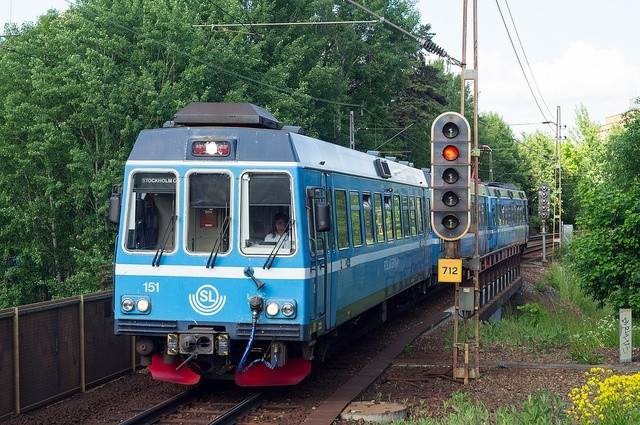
[542,121,563,251]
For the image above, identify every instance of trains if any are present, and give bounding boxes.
[115,101,529,387]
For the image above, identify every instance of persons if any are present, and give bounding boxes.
[263,214,290,250]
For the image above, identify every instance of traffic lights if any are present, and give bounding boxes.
[539,182,550,219]
[430,111,471,242]
